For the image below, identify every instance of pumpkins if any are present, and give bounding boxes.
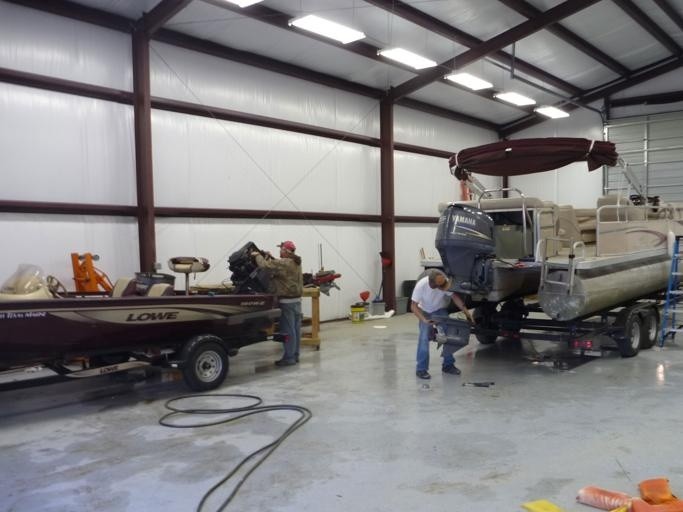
[658,235,683,348]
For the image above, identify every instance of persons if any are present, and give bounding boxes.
[411,268,475,379]
[259,241,302,365]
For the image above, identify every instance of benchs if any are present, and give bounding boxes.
[548,193,649,247]
[435,196,563,264]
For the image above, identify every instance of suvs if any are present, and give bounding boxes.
[355,302,370,318]
[350,305,367,324]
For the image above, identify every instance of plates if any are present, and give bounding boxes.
[188,282,321,351]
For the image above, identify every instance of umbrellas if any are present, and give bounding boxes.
[416,370,430,379]
[442,366,460,375]
[275,360,289,366]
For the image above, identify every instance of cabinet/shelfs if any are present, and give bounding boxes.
[188,282,321,351]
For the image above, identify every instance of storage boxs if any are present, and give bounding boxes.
[548,193,649,247]
[435,196,563,264]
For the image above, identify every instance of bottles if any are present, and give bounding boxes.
[429,270,450,291]
[276,241,295,252]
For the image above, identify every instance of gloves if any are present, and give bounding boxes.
[413,136,683,320]
[0,240,282,364]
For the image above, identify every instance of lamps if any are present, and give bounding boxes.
[377,2,437,69]
[494,70,536,106]
[534,81,570,119]
[288,2,366,44]
[443,41,493,90]
[228,1,258,8]
